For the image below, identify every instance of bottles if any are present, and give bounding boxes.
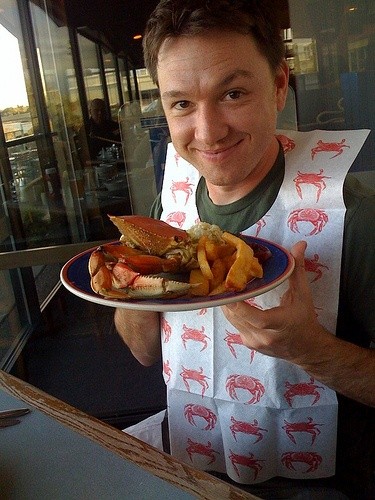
[284,44,295,76]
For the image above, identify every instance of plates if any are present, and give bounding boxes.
[59,236,295,312]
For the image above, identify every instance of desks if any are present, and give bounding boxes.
[0,367,263,500]
[3,174,131,243]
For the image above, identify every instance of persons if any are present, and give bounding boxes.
[114,0,375,500]
[74,98,132,241]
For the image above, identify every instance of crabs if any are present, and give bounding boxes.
[86,215,272,302]
